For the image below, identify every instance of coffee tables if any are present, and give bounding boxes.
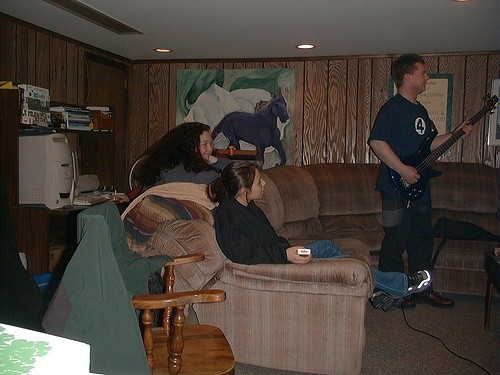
[483,250,500,331]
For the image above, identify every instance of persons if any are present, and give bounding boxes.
[368,54,473,310]
[208,160,435,312]
[139,122,231,196]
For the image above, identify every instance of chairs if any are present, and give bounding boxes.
[42,201,235,375]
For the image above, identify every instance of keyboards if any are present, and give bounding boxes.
[73,195,107,205]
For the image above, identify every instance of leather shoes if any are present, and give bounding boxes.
[417,290,455,307]
[400,298,416,308]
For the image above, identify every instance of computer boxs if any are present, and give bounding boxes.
[18,133,74,209]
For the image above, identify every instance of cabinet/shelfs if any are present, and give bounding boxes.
[0,88,126,277]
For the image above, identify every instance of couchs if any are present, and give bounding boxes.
[121,161,500,375]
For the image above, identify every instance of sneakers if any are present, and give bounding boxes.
[396,270,433,298]
[370,289,404,311]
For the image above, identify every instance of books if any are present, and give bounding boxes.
[51,105,93,131]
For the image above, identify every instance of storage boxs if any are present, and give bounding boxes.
[89,110,114,131]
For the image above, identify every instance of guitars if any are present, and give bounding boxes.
[387,94,498,201]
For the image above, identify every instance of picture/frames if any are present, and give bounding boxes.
[487,78,500,146]
